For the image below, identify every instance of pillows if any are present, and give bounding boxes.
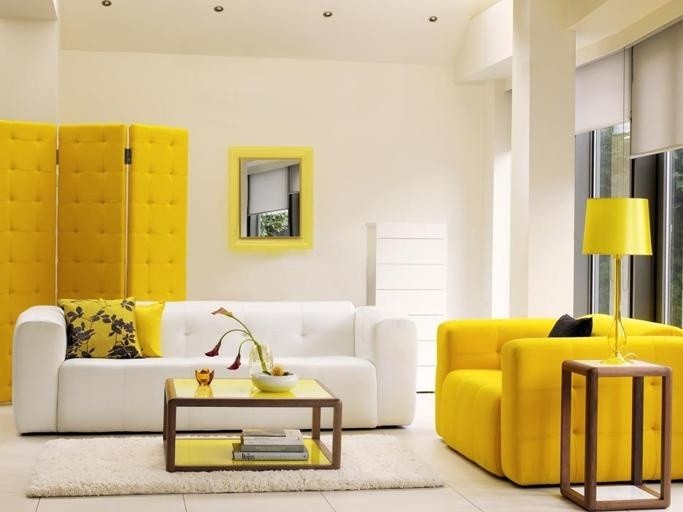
[58,298,167,359]
[548,315,593,340]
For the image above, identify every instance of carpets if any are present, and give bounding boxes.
[26,434,445,499]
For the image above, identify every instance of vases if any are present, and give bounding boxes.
[253,375,297,391]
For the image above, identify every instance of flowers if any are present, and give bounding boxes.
[205,307,294,376]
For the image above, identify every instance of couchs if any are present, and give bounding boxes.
[11,302,416,434]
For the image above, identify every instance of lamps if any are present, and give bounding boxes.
[582,196,655,368]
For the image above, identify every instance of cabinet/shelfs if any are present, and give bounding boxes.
[561,361,672,511]
[365,221,452,395]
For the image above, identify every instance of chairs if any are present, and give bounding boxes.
[436,315,682,485]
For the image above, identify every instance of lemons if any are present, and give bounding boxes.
[272,365,284,377]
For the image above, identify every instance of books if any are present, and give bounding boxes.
[230,428,309,461]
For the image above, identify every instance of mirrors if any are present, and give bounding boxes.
[228,145,314,249]
